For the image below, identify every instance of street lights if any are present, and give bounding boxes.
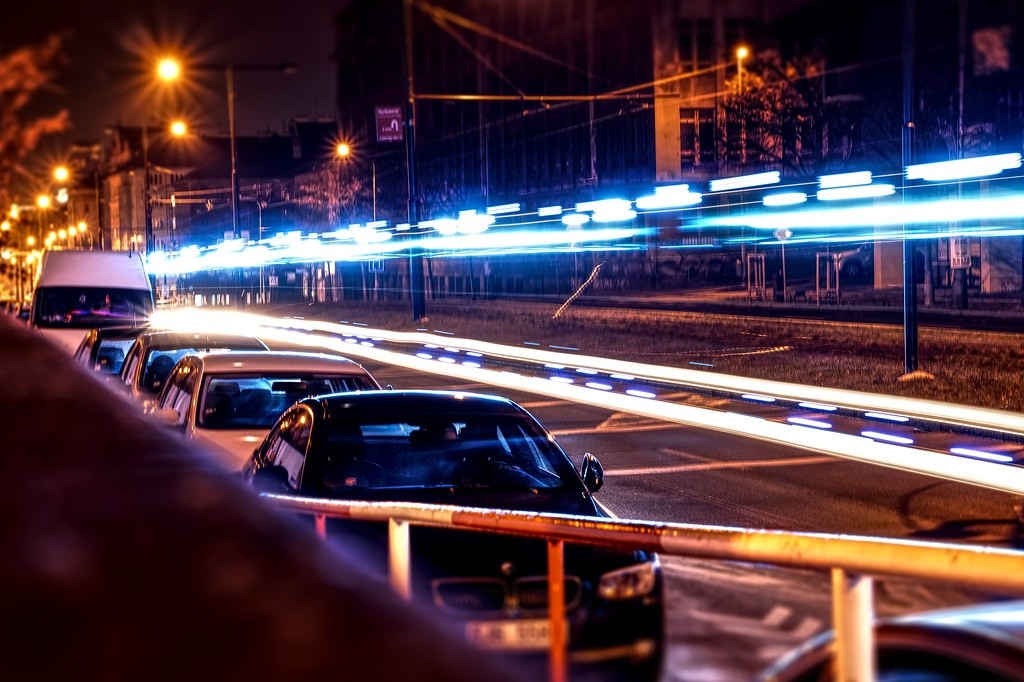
[337,144,381,299]
[157,58,240,236]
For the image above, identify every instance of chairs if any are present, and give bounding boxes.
[324,425,367,478]
[101,347,333,425]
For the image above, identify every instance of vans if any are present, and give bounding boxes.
[31,244,154,367]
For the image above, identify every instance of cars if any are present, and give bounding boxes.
[118,334,270,407]
[148,350,393,473]
[756,600,1024,682]
[73,328,144,386]
[242,386,666,681]
